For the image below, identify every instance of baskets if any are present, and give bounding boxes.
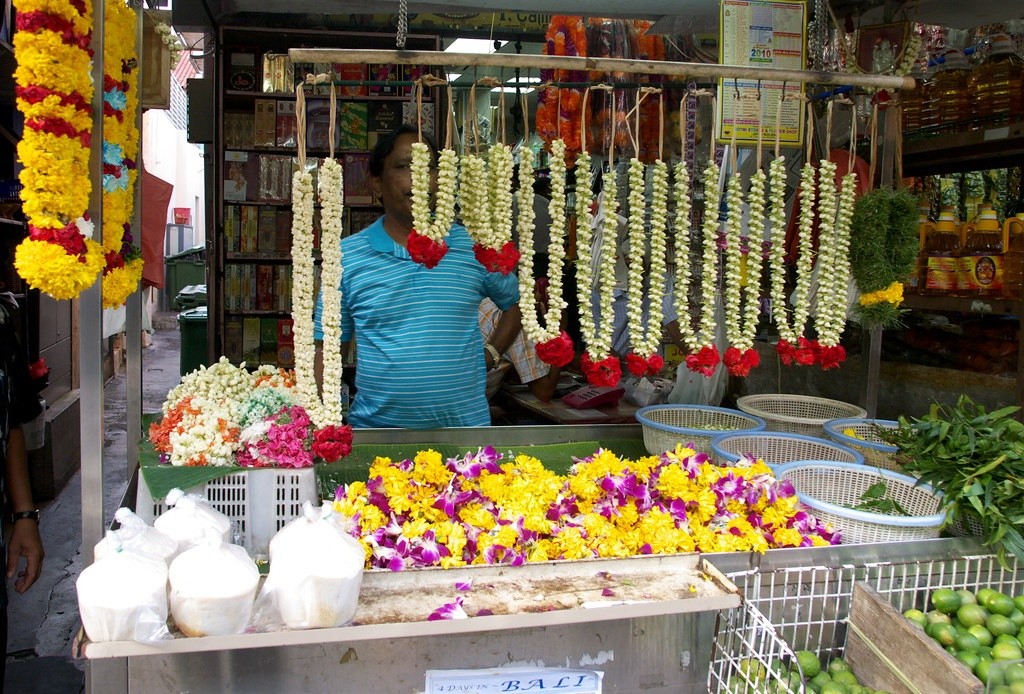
[635,393,1001,545]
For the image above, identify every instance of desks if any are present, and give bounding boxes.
[498,381,642,425]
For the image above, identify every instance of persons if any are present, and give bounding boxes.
[479,297,562,426]
[314,125,522,431]
[591,239,694,361]
[873,39,895,72]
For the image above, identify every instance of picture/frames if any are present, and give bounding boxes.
[852,19,908,95]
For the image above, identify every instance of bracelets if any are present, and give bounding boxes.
[484,344,502,369]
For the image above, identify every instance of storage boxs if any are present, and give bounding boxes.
[134,466,317,556]
[223,53,436,364]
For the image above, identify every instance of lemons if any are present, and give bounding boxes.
[719,650,892,694]
[896,588,1024,694]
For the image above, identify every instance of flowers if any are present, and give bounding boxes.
[11,1,353,468]
[406,138,857,389]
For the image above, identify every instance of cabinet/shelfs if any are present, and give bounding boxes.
[217,24,442,370]
[29,389,80,501]
[28,289,72,409]
[876,119,1023,420]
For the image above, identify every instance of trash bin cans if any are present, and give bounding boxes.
[177,305,208,383]
[164,245,208,311]
[174,283,208,311]
